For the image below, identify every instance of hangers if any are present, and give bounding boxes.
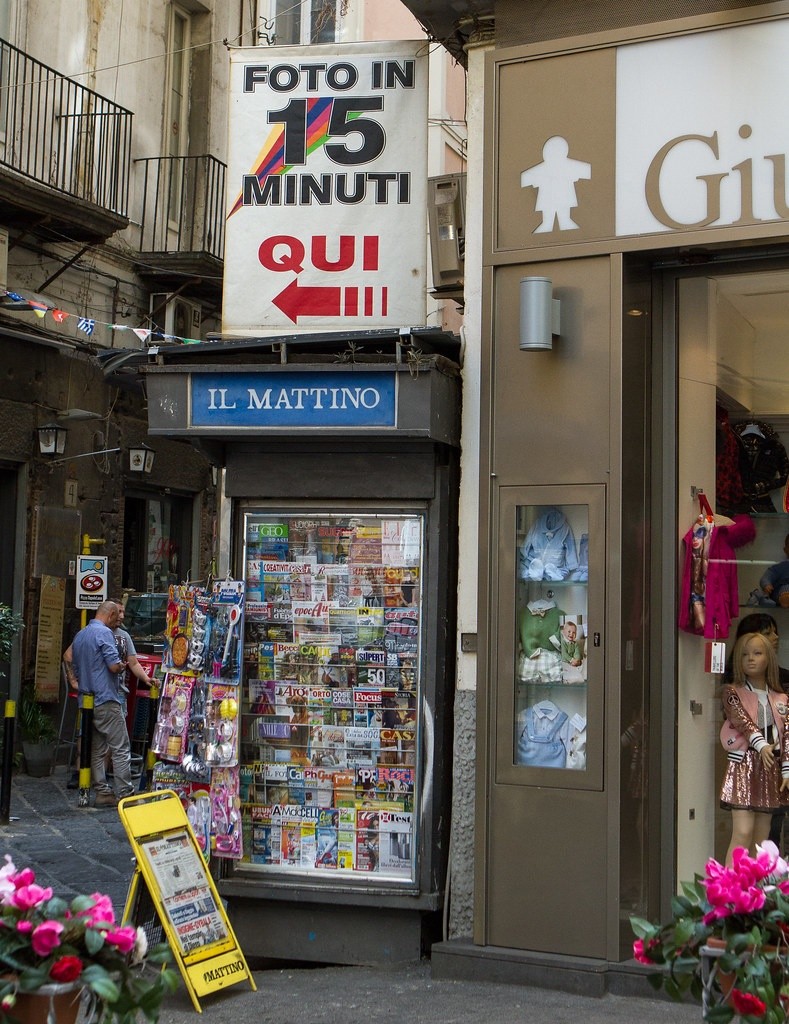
[741,411,766,439]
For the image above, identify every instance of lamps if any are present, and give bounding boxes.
[518,277,561,352]
[34,422,72,461]
[209,462,226,487]
[127,442,158,479]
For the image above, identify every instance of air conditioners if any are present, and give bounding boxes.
[148,291,203,345]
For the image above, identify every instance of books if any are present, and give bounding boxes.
[241,519,419,876]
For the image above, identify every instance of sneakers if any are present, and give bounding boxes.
[95,794,116,807]
[117,792,146,807]
[67,774,79,789]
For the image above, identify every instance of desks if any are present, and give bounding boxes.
[106,652,162,780]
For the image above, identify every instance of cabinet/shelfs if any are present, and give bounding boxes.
[717,513,789,612]
[234,496,426,899]
[498,484,604,793]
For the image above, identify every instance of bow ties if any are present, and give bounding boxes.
[546,532,553,537]
[569,641,574,644]
[532,608,545,617]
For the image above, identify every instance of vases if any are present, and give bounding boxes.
[3,980,82,1024]
[708,936,789,1017]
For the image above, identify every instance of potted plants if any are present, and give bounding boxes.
[14,683,58,778]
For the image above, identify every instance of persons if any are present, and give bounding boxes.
[562,622,581,668]
[722,534,789,869]
[62,598,160,808]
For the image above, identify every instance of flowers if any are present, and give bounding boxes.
[626,838,789,1024]
[0,853,183,1024]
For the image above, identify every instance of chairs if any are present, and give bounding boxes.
[51,662,79,774]
